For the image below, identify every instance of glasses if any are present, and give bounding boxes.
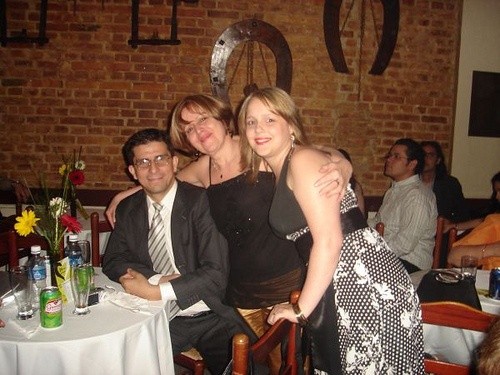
[388,152,408,161]
[133,153,174,169]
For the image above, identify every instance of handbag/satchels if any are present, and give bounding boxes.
[418,268,482,314]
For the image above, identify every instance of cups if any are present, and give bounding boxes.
[70,266,91,316]
[461,255,478,280]
[10,265,33,321]
[77,239,90,267]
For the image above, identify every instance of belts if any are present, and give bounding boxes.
[177,310,214,319]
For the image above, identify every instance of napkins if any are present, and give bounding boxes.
[111,290,148,312]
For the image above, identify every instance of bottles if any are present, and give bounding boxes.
[23,245,47,310]
[63,234,83,279]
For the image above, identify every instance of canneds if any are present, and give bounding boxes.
[40,286,62,329]
[488,267,500,300]
[75,264,95,295]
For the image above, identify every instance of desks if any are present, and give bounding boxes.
[66,210,112,254]
[0,264,177,375]
[410,267,500,366]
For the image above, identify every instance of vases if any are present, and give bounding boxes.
[45,241,62,284]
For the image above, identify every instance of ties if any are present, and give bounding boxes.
[148,202,181,322]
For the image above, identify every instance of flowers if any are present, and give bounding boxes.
[14,144,86,250]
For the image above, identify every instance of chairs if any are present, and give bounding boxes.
[175,216,500,375]
[0,211,114,274]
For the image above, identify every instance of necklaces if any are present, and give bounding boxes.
[213,164,222,178]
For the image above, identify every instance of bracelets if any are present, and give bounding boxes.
[292,301,306,322]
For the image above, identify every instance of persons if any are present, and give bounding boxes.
[237,87,424,375]
[421,140,470,222]
[337,149,365,216]
[103,94,353,375]
[443,172,500,270]
[472,317,500,375]
[103,128,243,375]
[366,138,438,290]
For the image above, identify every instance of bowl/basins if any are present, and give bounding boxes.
[479,298,500,315]
